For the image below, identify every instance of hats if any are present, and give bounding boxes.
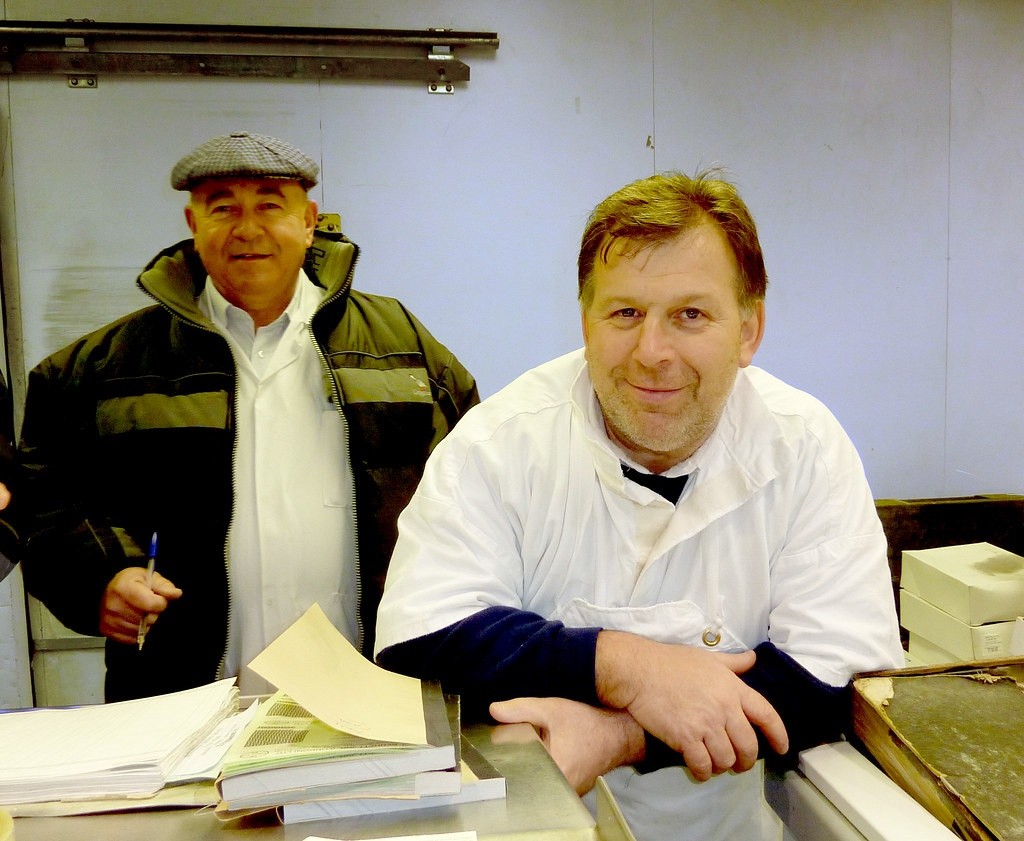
[171,130,320,190]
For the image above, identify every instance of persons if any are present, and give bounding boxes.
[373,161,905,841]
[0,128,482,705]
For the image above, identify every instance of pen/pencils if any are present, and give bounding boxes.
[136,531,158,651]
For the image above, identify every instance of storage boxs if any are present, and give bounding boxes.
[900,540,1024,665]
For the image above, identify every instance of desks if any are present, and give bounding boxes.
[0,692,604,841]
